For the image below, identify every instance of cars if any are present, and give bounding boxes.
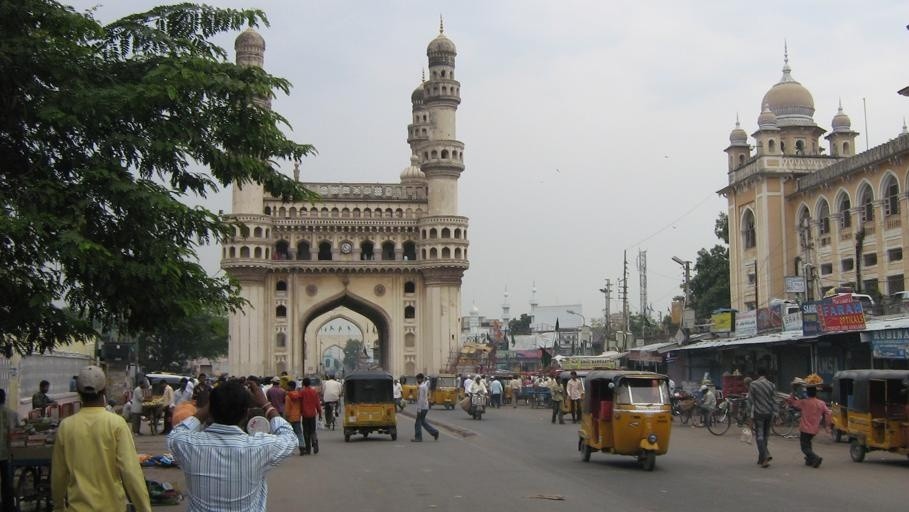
[883,289,909,316]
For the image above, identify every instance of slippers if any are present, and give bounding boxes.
[761,463,771,468]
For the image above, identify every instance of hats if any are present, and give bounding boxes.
[270,376,281,383]
[467,375,471,378]
[490,376,494,379]
[77,365,106,394]
[699,384,708,392]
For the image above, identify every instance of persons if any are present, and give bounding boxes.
[456,370,587,427]
[785,385,835,469]
[745,366,777,468]
[699,384,717,428]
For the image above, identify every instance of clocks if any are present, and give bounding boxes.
[340,240,353,254]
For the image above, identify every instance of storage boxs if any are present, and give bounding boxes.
[9,444,54,467]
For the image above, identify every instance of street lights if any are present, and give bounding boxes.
[566,309,586,355]
[671,256,692,307]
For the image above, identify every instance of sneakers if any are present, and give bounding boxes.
[435,429,440,440]
[411,439,422,442]
[312,439,319,454]
[300,444,311,456]
[805,453,823,468]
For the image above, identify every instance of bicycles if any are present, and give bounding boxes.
[323,402,338,431]
[147,407,163,435]
[676,390,794,439]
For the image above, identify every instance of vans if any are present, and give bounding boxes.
[145,372,197,396]
[838,293,876,317]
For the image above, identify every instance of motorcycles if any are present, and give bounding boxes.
[557,368,674,472]
[827,367,908,465]
[341,369,514,442]
